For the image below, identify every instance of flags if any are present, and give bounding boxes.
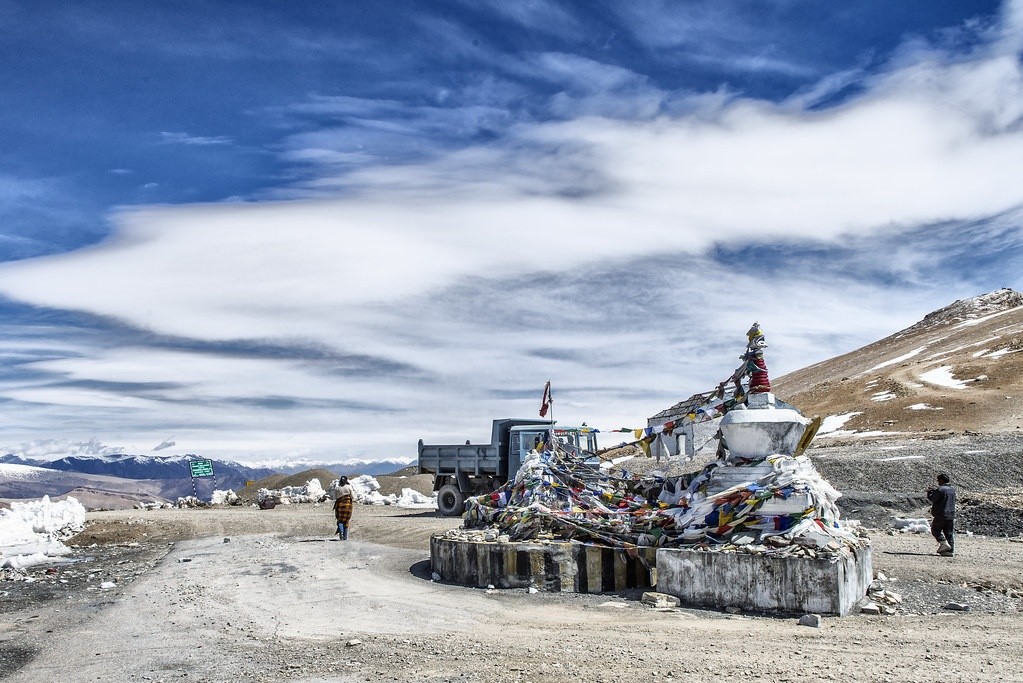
[618,360,761,464]
[539,382,550,417]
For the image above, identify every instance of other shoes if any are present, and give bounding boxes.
[937,541,951,553]
[940,551,954,557]
[339,533,343,540]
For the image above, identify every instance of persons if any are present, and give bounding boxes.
[334,476,354,540]
[927,473,955,557]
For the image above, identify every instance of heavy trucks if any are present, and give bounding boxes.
[417,418,601,517]
